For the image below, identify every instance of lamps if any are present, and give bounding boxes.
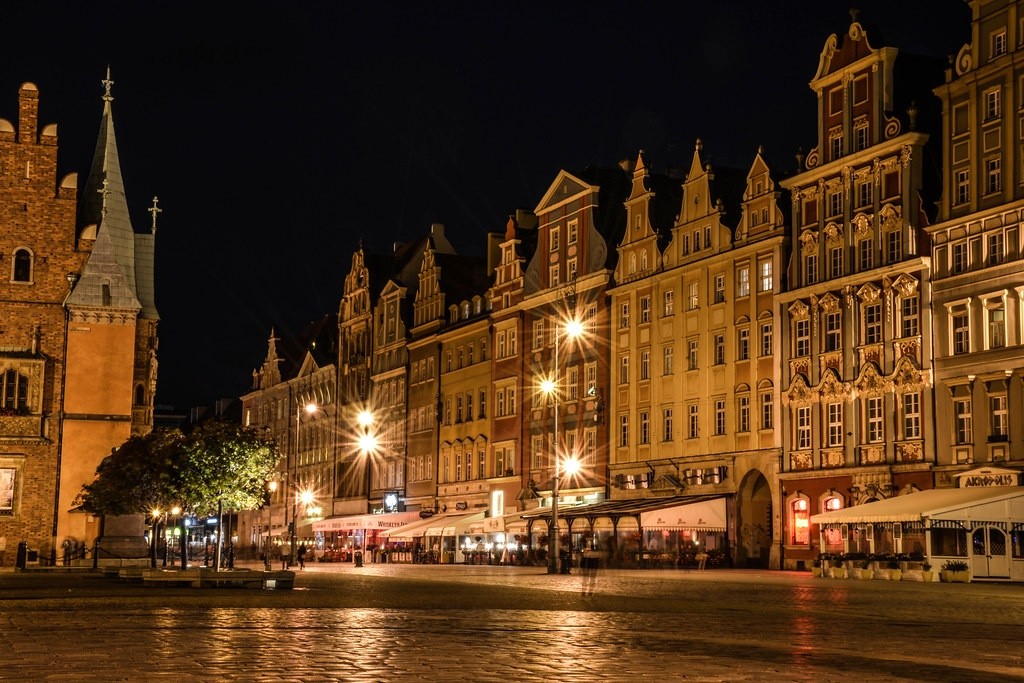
[66,273,76,290]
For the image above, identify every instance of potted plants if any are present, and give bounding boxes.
[507,534,569,566]
[942,560,971,582]
[492,550,503,565]
[385,548,417,564]
[471,550,488,564]
[632,548,718,571]
[812,553,932,582]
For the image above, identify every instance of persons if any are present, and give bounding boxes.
[578,531,600,596]
[61,536,78,567]
[280,542,292,570]
[297,542,306,570]
[696,540,708,570]
[251,540,256,559]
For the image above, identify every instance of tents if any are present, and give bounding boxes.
[311,496,727,564]
[809,486,1024,582]
